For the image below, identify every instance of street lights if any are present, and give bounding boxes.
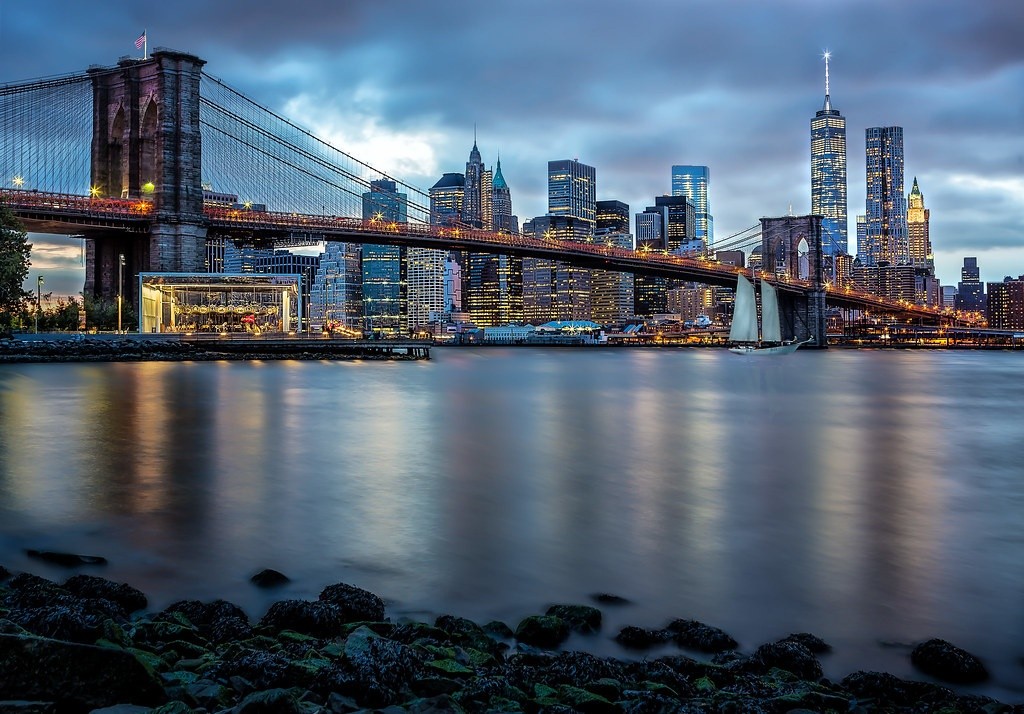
[38,274,44,305]
[118,253,126,333]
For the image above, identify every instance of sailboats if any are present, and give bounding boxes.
[726,265,815,357]
